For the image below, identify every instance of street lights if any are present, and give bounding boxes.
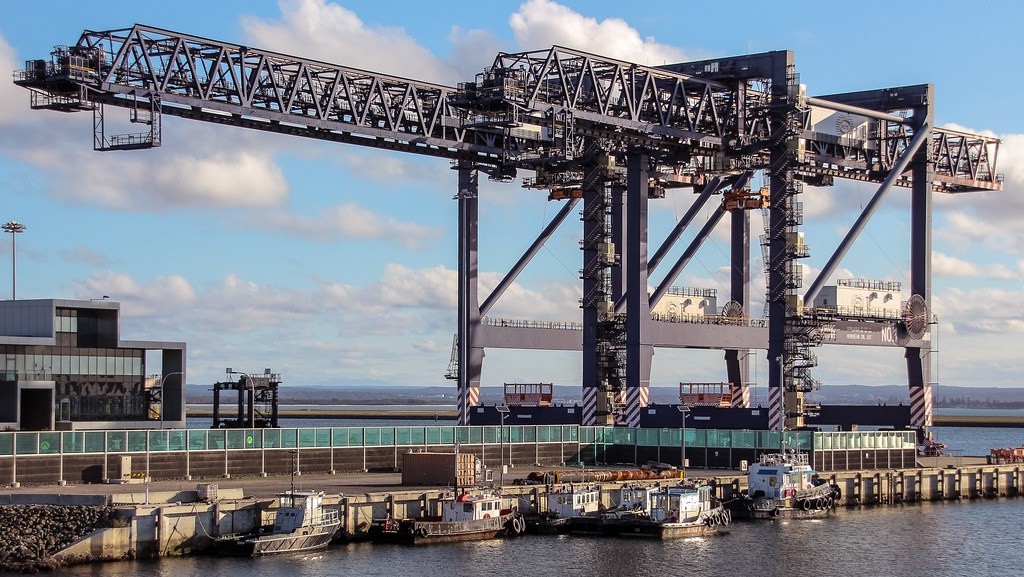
[158,372,185,430]
[1,220,28,299]
[229,371,255,429]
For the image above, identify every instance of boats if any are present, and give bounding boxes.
[597,476,733,539]
[512,481,612,533]
[369,485,529,549]
[213,491,341,562]
[725,352,840,519]
